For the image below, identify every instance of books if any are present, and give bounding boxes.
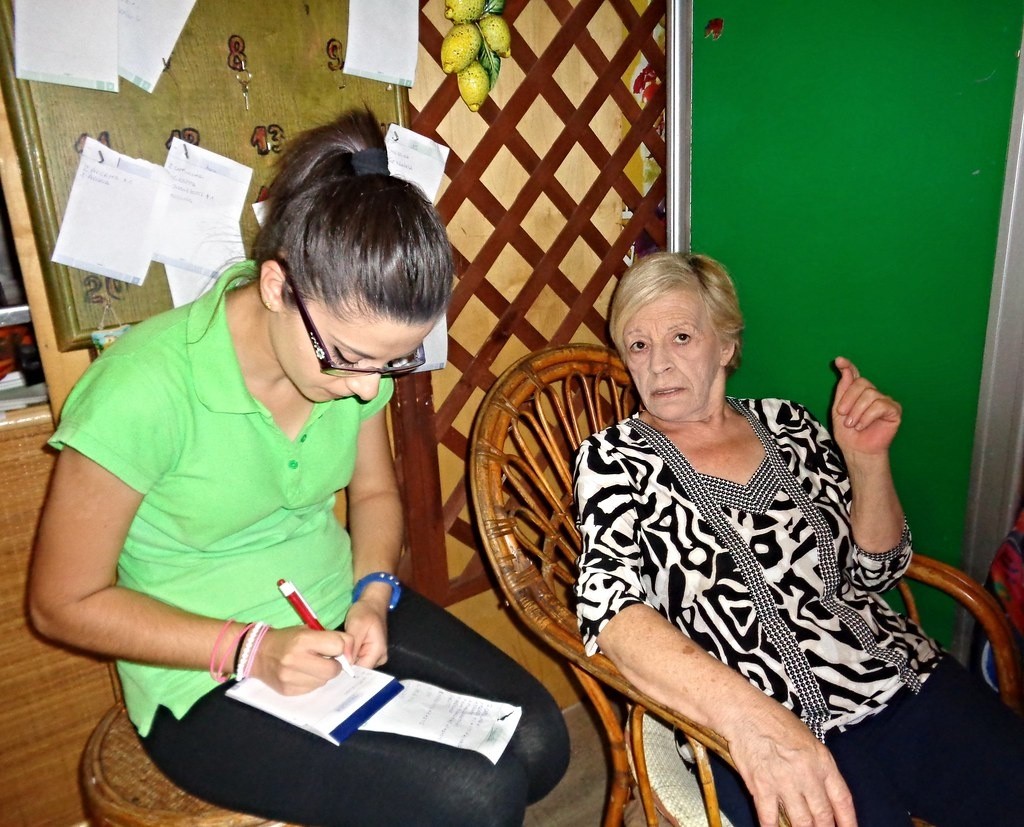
[225,665,405,746]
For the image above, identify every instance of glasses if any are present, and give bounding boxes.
[276,255,426,377]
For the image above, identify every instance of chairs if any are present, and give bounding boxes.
[469,345,1017,826]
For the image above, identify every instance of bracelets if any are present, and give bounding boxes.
[210,620,272,682]
[352,572,401,611]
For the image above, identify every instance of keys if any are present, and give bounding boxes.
[242,81,249,110]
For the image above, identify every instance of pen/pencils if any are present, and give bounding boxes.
[276,577,356,681]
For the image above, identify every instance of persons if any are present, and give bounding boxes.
[570,253,1024,826]
[28,109,571,826]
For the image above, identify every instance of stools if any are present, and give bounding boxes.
[77,695,303,826]
